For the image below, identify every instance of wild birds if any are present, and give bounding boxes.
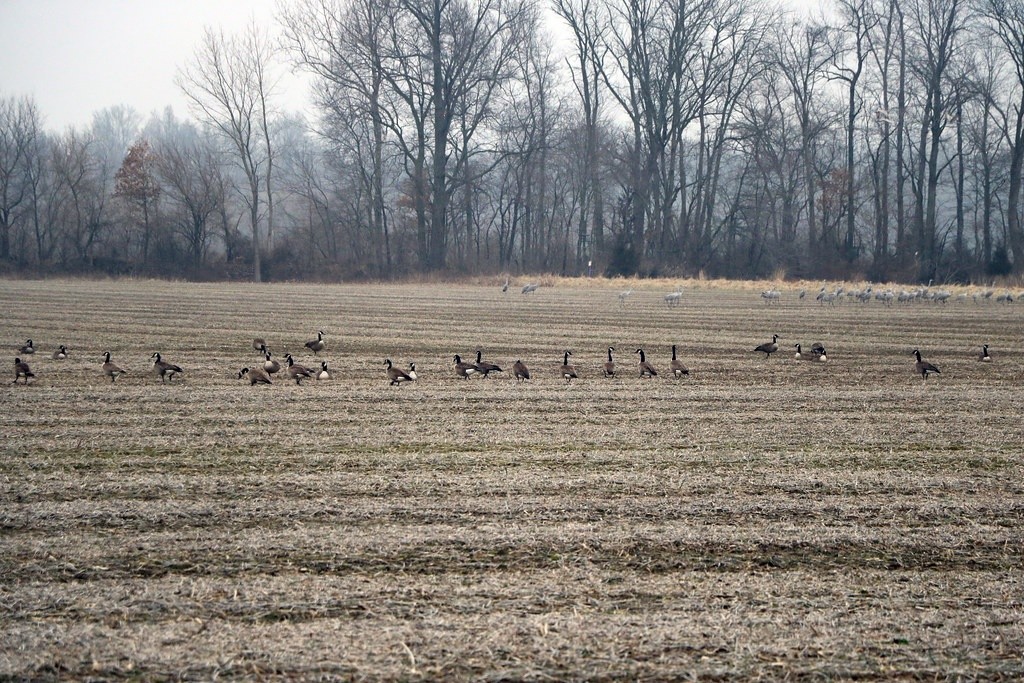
[303,331,326,356]
[521,278,540,295]
[634,348,658,379]
[502,277,510,295]
[472,351,504,380]
[793,342,828,364]
[12,357,35,383]
[977,344,994,363]
[150,352,184,383]
[452,355,482,381]
[663,284,687,309]
[760,285,786,308]
[101,351,128,383]
[237,351,316,384]
[754,333,781,359]
[798,286,806,302]
[382,359,418,386]
[51,345,69,360]
[815,278,1024,308]
[670,344,690,381]
[910,349,941,381]
[617,287,635,309]
[253,338,269,356]
[18,339,35,355]
[560,351,579,384]
[602,347,617,379]
[315,361,333,381]
[512,360,530,383]
